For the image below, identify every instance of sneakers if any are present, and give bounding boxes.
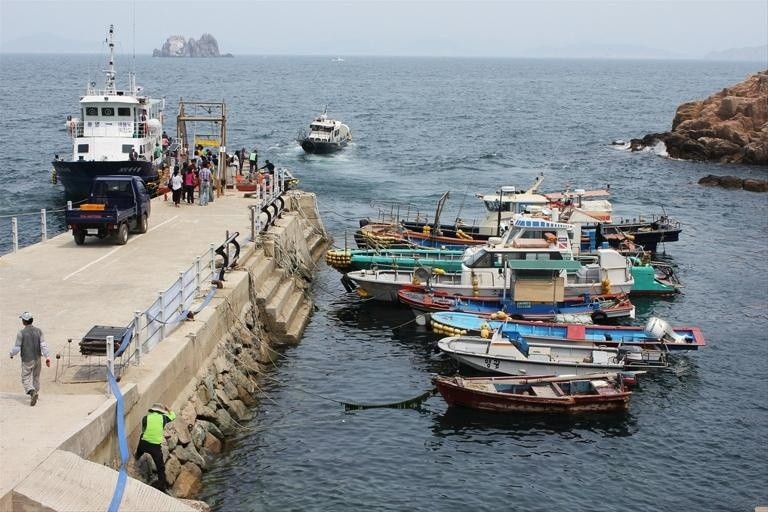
[30,390,39,406]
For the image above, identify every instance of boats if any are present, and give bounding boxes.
[430,371,634,417]
[322,173,686,298]
[427,310,707,350]
[393,283,639,331]
[51,24,177,196]
[296,105,352,153]
[438,323,670,376]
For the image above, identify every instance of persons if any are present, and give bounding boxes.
[10,312,50,406]
[129,149,138,161]
[133,403,176,494]
[249,150,257,173]
[139,110,147,133]
[154,131,249,208]
[258,160,274,175]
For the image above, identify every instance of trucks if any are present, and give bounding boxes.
[65,175,150,245]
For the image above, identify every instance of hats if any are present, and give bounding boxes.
[148,402,167,414]
[19,311,34,321]
